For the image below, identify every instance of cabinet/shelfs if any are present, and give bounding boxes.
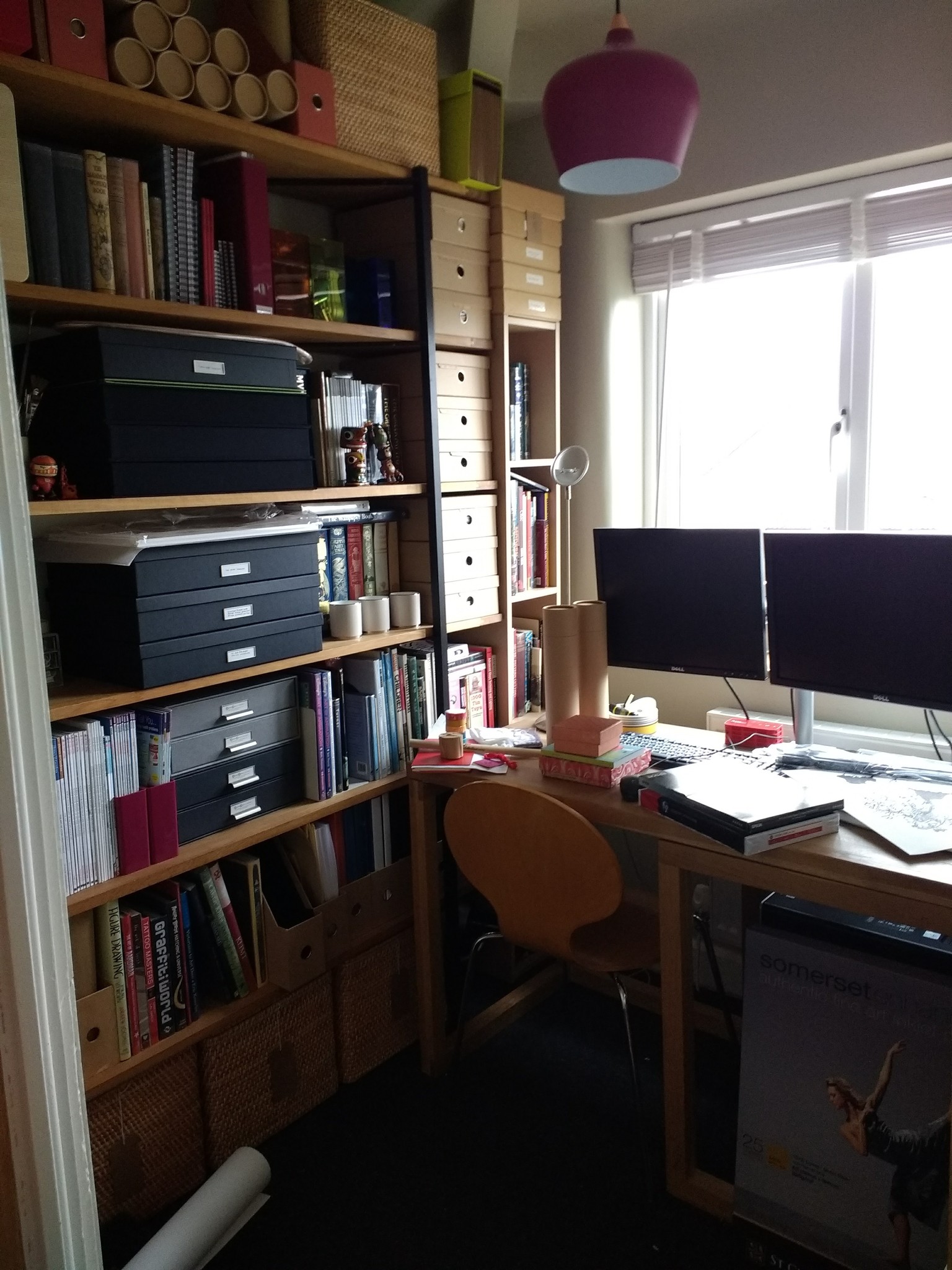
[430,186,559,735]
[2,1,432,1234]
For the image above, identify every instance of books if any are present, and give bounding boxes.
[509,363,532,462]
[94,855,268,1062]
[316,521,400,615]
[510,470,549,596]
[17,142,239,309]
[512,615,546,718]
[447,645,497,728]
[298,646,437,801]
[540,742,643,768]
[307,371,399,487]
[51,706,172,897]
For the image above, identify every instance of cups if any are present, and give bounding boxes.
[329,600,363,640]
[388,591,422,629]
[357,595,390,634]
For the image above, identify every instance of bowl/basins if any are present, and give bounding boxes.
[609,702,658,736]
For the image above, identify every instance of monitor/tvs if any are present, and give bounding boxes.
[593,528,952,713]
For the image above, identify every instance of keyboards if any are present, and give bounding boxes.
[621,732,798,777]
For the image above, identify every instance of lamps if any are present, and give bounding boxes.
[548,446,590,603]
[542,0,698,199]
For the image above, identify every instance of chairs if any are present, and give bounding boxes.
[438,779,736,1183]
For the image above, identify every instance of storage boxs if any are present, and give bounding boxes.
[23,320,310,501]
[725,715,783,748]
[46,514,323,691]
[539,714,651,788]
[489,180,566,324]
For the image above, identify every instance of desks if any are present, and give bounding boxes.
[402,741,952,1237]
